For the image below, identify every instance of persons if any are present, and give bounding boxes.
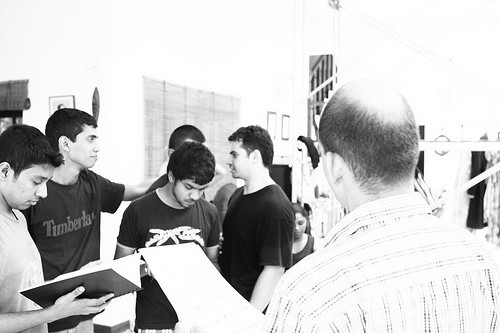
[113,141,221,333]
[292,202,326,267]
[21,107,160,333]
[296,139,346,240]
[174,76,500,333]
[145,124,227,200]
[0,124,116,333]
[222,126,296,316]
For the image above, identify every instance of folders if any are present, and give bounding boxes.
[21,253,142,310]
